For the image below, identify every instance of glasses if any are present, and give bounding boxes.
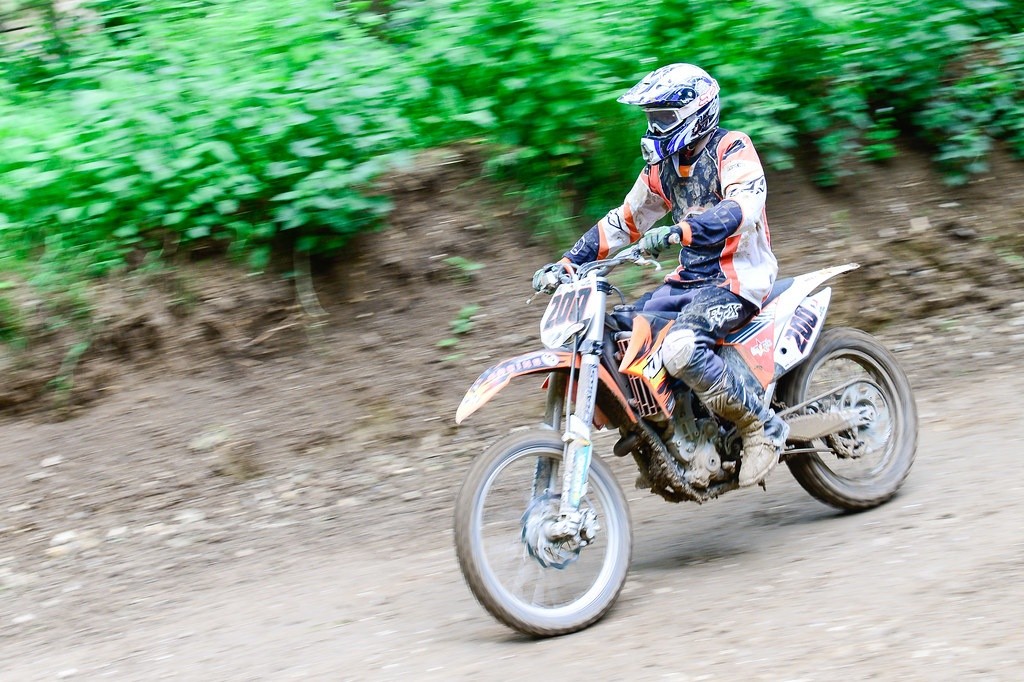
[641,107,683,133]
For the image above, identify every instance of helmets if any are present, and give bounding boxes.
[616,63,721,164]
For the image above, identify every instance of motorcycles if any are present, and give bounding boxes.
[452,231,921,637]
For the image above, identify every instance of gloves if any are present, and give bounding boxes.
[643,224,683,259]
[533,263,566,295]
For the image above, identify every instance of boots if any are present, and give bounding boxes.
[694,360,791,486]
[636,474,651,490]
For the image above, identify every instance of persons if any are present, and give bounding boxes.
[531,62,791,489]
